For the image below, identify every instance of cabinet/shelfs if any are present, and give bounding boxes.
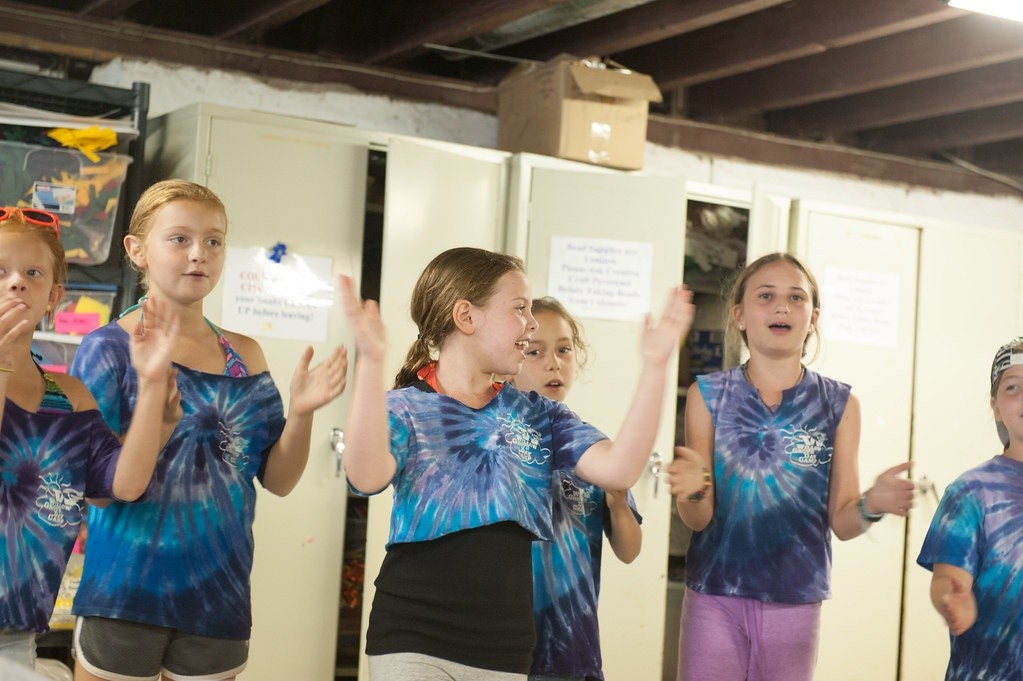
[506,153,792,681]
[135,102,512,681]
[789,199,1023,681]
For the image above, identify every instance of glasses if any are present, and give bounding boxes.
[0,207,61,240]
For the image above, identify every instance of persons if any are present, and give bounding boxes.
[68,178,349,681]
[495,295,643,680]
[339,246,696,680]
[661,252,916,681]
[0,205,180,681]
[918,333,1023,680]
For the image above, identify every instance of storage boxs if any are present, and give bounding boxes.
[496,52,663,170]
[0,102,140,265]
[40,282,118,337]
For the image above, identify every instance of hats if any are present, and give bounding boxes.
[991,335,1023,447]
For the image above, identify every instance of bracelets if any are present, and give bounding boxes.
[0,367,15,376]
[858,492,884,524]
[689,464,710,502]
